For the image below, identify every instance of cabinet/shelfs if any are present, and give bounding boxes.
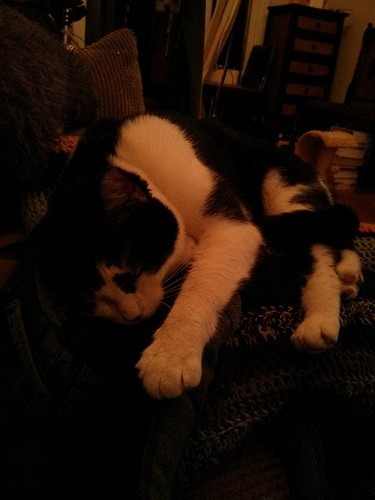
[256,1,351,129]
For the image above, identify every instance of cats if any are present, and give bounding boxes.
[1,110,365,399]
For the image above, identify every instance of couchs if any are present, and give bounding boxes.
[2,3,374,500]
